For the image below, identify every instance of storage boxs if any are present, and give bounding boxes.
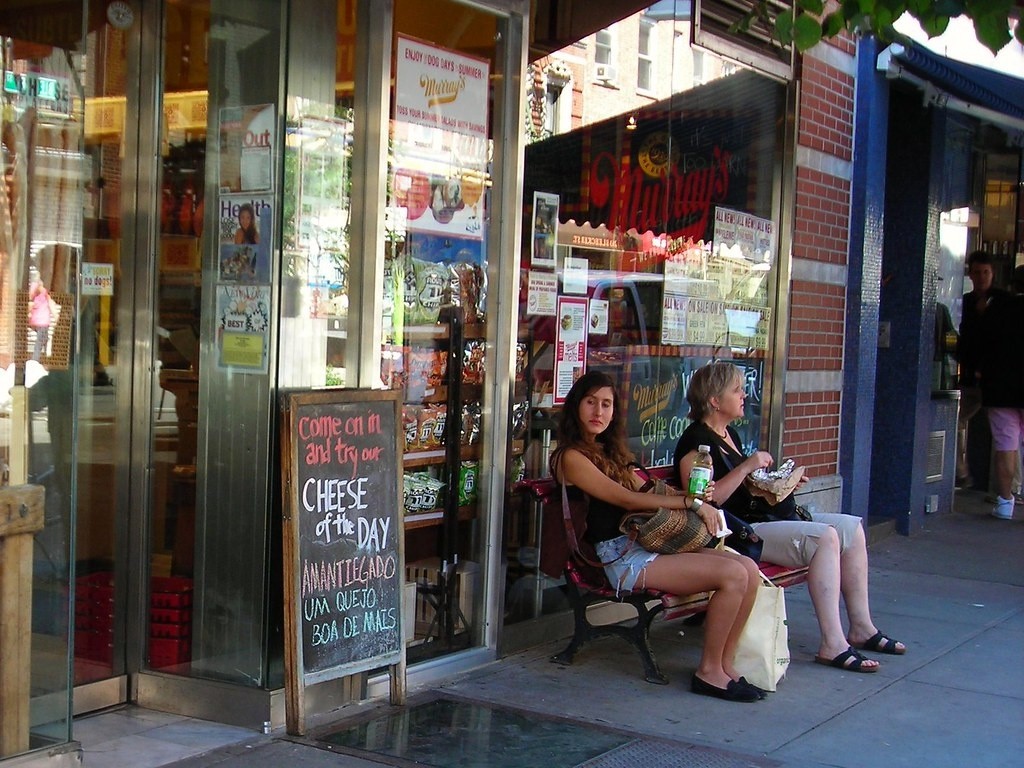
[64,573,193,670]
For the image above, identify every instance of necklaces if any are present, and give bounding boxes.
[719,430,727,439]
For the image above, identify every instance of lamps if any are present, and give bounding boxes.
[626,114,639,129]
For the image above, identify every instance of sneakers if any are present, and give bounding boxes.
[955,474,973,490]
[990,493,1015,520]
[985,490,1023,505]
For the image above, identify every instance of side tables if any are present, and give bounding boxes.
[327,307,531,622]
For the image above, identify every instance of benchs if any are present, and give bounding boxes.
[508,466,811,685]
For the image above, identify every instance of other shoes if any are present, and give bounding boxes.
[691,673,761,702]
[735,676,769,700]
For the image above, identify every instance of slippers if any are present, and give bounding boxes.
[846,630,906,654]
[815,646,878,672]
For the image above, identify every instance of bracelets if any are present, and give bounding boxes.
[684,495,704,512]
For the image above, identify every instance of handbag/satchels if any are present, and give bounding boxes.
[746,491,813,522]
[619,462,713,555]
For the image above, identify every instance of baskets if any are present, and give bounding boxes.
[63,570,194,673]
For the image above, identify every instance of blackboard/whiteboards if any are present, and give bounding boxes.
[618,345,772,468]
[282,389,407,688]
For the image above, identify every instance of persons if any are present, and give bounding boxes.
[549,371,767,703]
[935,250,1024,520]
[233,202,260,245]
[673,362,907,672]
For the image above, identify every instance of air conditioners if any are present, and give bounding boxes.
[596,64,614,81]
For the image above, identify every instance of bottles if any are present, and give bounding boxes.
[777,459,795,478]
[688,444,713,501]
